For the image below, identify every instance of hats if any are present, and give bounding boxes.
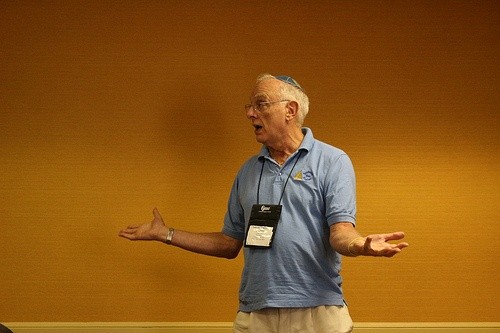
[275,76,304,91]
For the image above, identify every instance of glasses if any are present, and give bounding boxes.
[244,99,291,113]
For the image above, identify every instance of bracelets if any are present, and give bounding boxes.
[165,227,174,244]
[343,236,364,256]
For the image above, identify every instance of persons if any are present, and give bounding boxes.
[118,75,409,333]
